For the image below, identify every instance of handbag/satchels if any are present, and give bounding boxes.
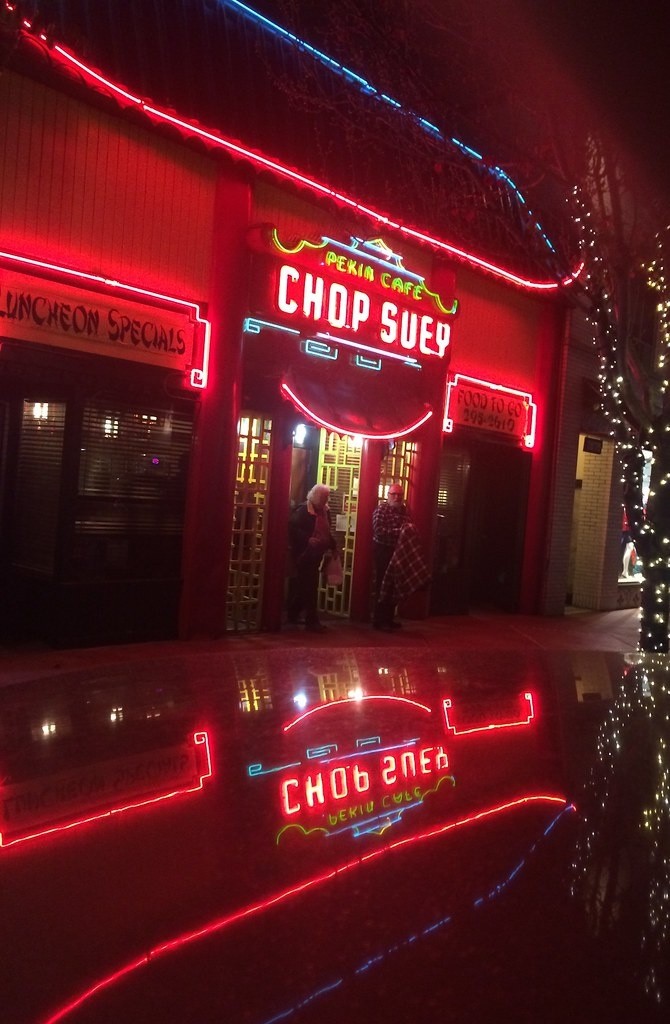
[326,555,343,586]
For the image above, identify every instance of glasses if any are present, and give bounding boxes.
[388,492,405,498]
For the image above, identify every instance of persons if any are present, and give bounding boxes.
[372,484,416,631]
[285,483,340,631]
[621,508,633,578]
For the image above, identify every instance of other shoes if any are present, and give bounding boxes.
[387,621,401,629]
[372,621,396,633]
[306,618,328,632]
[288,615,305,624]
